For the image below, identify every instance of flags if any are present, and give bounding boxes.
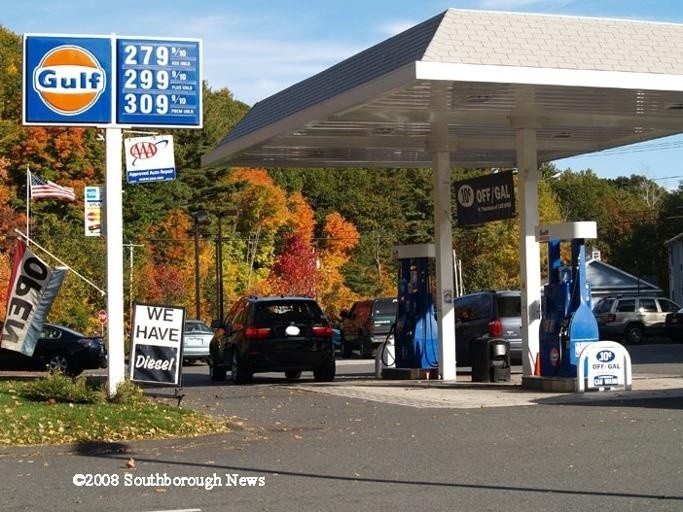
[1,236,68,358]
[29,171,76,202]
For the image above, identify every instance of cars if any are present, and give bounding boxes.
[182,319,215,367]
[0,322,106,380]
[454,290,523,369]
[664,307,683,345]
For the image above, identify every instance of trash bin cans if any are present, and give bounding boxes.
[470,338,511,383]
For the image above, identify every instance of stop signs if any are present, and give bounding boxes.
[98,310,107,325]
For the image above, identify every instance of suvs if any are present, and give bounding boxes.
[593,294,681,346]
[338,296,398,359]
[207,293,335,384]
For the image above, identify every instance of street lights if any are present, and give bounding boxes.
[216,207,237,319]
[189,210,207,318]
